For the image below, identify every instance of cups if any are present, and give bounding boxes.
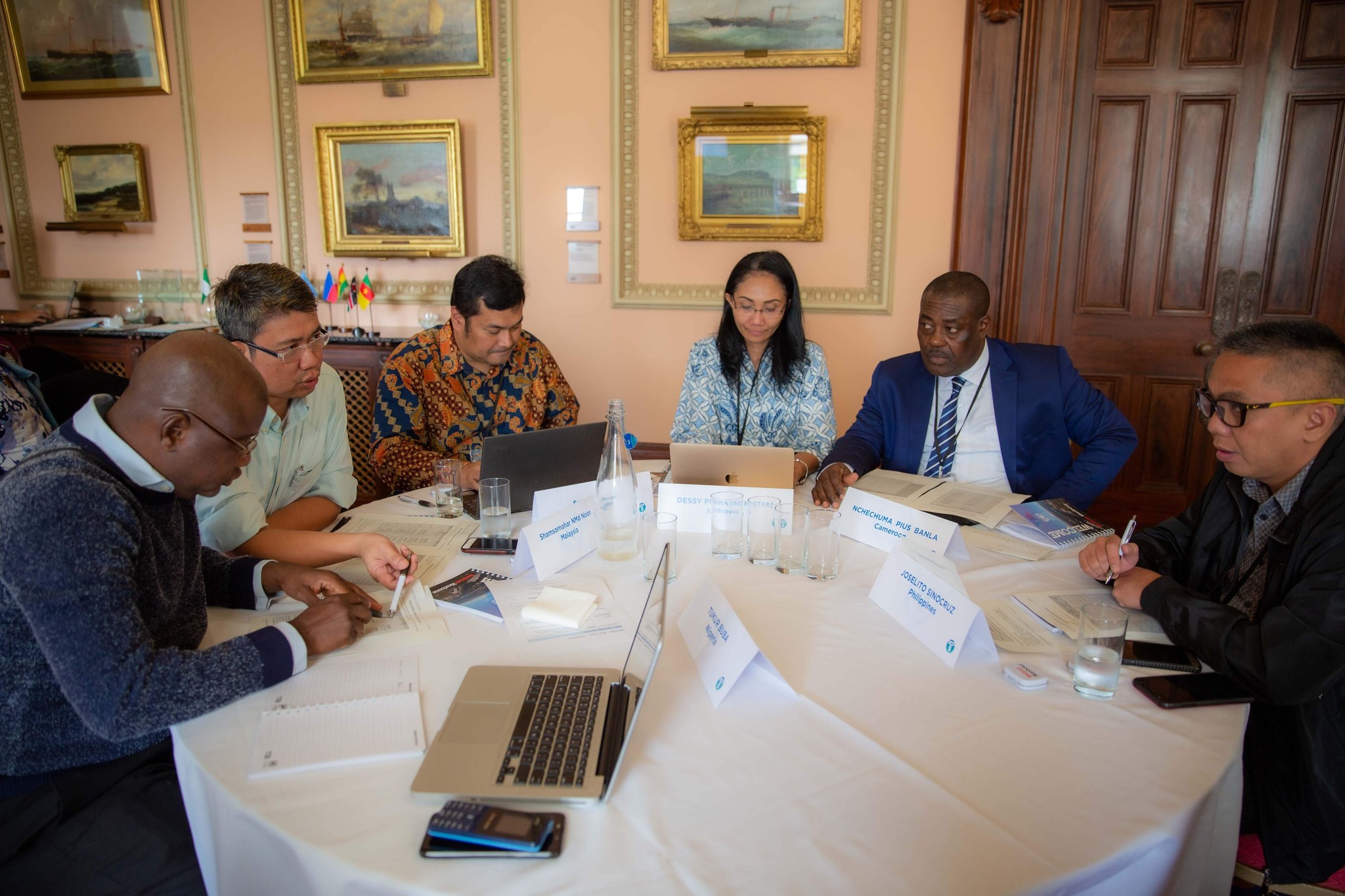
[432,459,463,519]
[1071,602,1130,701]
[477,478,512,539]
[774,503,809,574]
[747,495,781,566]
[711,491,743,560]
[642,512,677,584]
[805,509,842,582]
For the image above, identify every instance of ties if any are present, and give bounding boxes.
[923,375,966,479]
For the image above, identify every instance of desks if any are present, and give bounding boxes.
[166,460,1242,896]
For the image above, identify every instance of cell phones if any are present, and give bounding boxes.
[1092,637,1203,672]
[419,813,566,859]
[424,800,556,852]
[461,537,517,556]
[1132,671,1256,710]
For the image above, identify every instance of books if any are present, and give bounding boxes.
[429,566,514,623]
[998,497,1116,551]
[248,652,426,782]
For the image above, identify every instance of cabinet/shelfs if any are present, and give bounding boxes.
[0,318,432,513]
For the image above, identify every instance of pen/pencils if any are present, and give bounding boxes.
[1104,515,1136,583]
[362,608,386,618]
[397,494,435,508]
[387,552,411,617]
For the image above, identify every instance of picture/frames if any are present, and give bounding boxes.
[0,0,170,100]
[678,116,828,244]
[286,0,497,86]
[651,0,863,71]
[52,142,152,224]
[312,117,467,258]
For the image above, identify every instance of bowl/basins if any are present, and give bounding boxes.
[418,304,451,330]
[200,302,219,325]
[120,301,148,322]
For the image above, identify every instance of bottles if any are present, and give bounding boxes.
[595,399,639,562]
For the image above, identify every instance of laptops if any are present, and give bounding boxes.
[669,443,794,489]
[446,420,607,520]
[412,542,671,803]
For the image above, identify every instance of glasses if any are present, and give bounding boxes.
[733,293,787,316]
[1194,388,1345,428]
[162,408,259,460]
[229,327,329,363]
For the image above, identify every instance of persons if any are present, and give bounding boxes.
[0,328,382,896]
[194,263,419,591]
[368,255,578,494]
[671,251,838,489]
[1078,322,1345,890]
[0,308,59,476]
[811,271,1140,514]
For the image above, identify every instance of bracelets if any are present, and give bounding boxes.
[793,459,808,485]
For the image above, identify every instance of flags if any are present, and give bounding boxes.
[300,264,375,313]
[201,264,211,304]
